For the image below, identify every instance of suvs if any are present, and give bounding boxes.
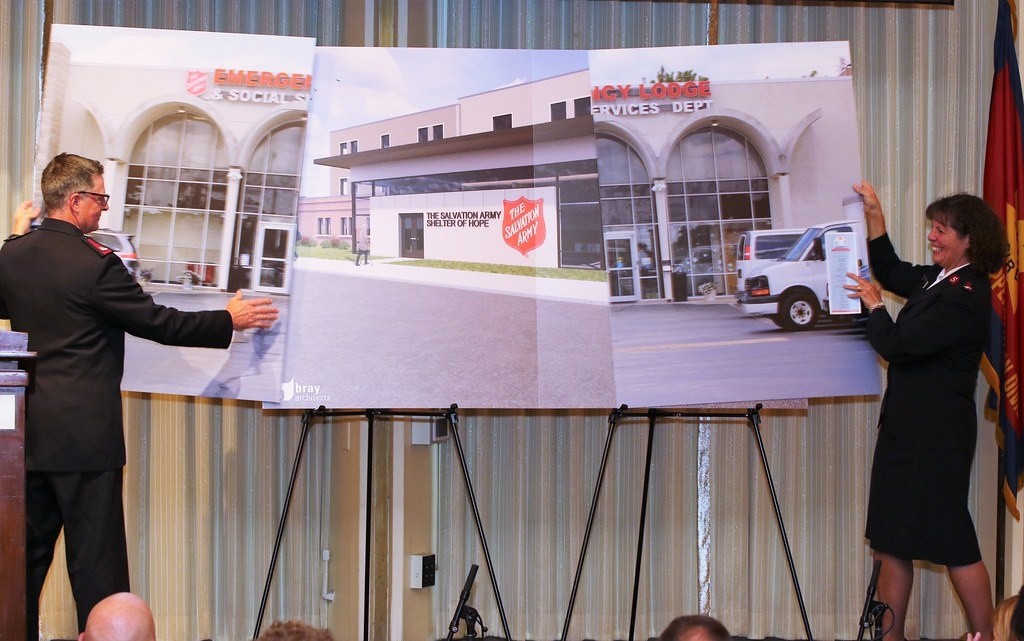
[88,228,140,281]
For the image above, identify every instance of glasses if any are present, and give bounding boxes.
[78,191,110,206]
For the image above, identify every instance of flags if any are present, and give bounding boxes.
[981,0,1024,521]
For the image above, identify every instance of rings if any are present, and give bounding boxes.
[858,287,861,291]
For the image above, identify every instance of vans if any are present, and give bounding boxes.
[737,219,868,331]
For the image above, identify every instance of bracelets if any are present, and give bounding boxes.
[9,234,20,238]
[869,301,885,314]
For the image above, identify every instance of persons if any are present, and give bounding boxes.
[78,592,156,641]
[659,615,729,641]
[638,243,649,299]
[355,224,369,266]
[967,585,1024,641]
[0,154,279,641]
[844,179,1006,641]
